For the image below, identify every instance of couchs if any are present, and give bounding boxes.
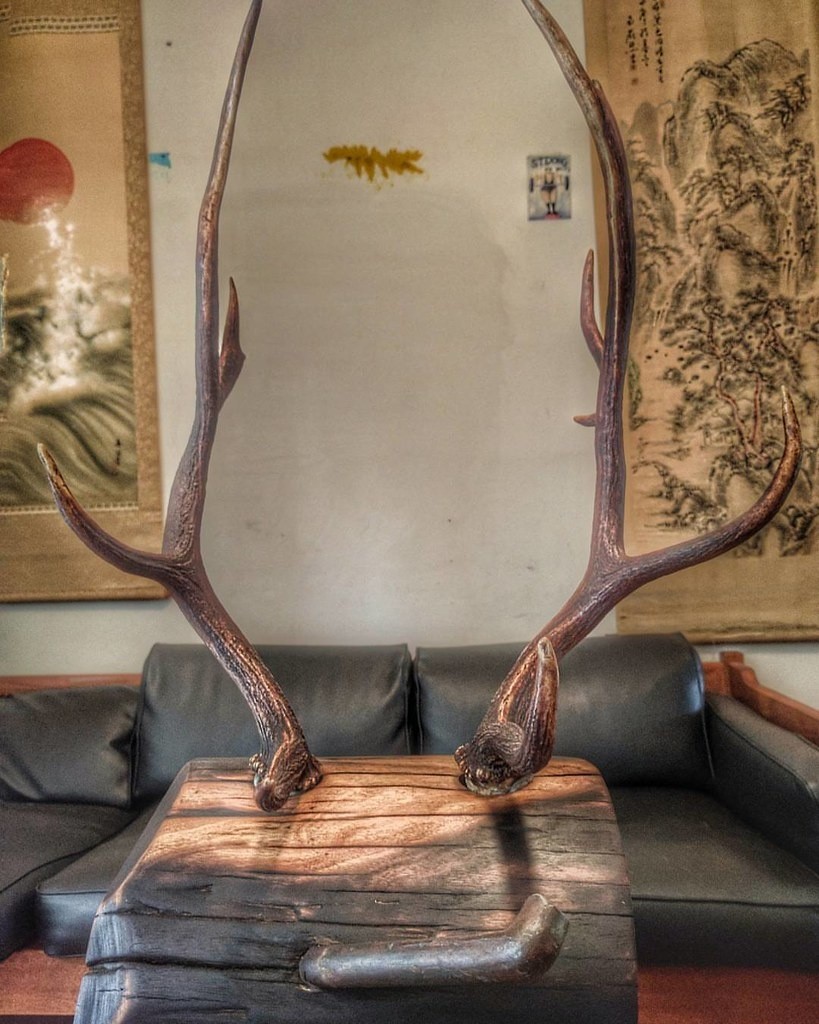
[0,630,818,1023]
